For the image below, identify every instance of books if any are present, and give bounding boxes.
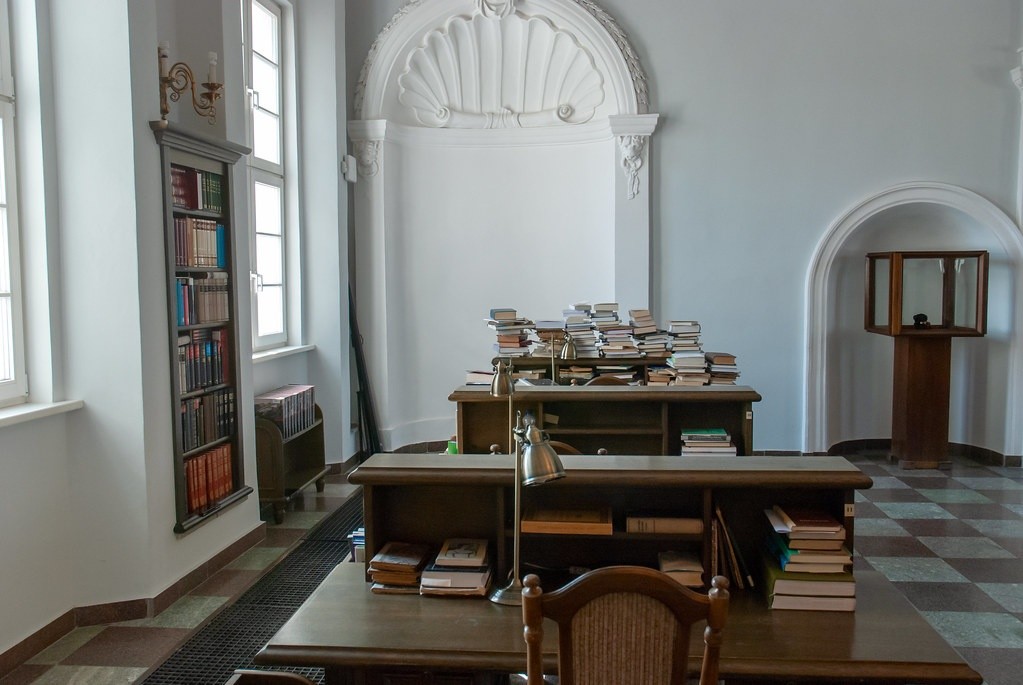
[254,384,316,438]
[626,505,703,535]
[764,499,857,611]
[712,504,755,591]
[171,163,225,214]
[657,551,705,587]
[181,388,235,452]
[420,537,493,597]
[175,271,230,326]
[173,217,225,268]
[465,303,741,386]
[184,439,239,514]
[177,327,231,394]
[347,528,365,562]
[367,541,432,594]
[680,419,737,457]
[520,501,613,536]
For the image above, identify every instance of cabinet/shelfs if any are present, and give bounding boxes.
[147,121,253,534]
[256,403,329,524]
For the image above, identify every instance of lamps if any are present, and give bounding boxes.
[550,328,577,386]
[490,360,514,454]
[491,410,567,606]
[157,38,224,124]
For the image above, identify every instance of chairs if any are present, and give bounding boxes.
[521,565,729,685]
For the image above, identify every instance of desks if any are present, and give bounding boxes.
[492,356,668,385]
[253,557,983,685]
[445,389,762,454]
[348,451,873,581]
